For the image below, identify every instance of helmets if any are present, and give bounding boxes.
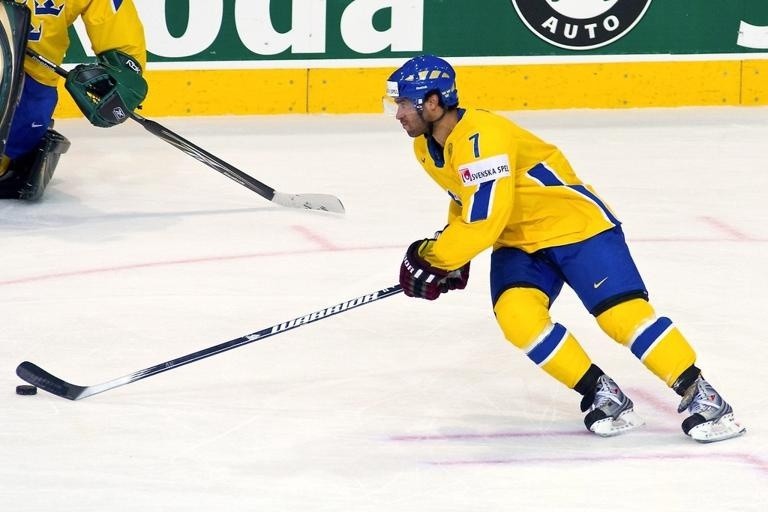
[386,55,459,113]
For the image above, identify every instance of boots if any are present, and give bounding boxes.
[677,372,733,437]
[579,373,634,433]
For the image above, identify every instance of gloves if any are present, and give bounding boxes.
[64,49,148,129]
[434,224,471,294]
[399,238,451,301]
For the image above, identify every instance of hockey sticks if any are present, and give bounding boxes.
[16,283,404,400]
[25,47,346,216]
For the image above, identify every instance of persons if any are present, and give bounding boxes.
[388,56,745,440]
[0,1,149,203]
